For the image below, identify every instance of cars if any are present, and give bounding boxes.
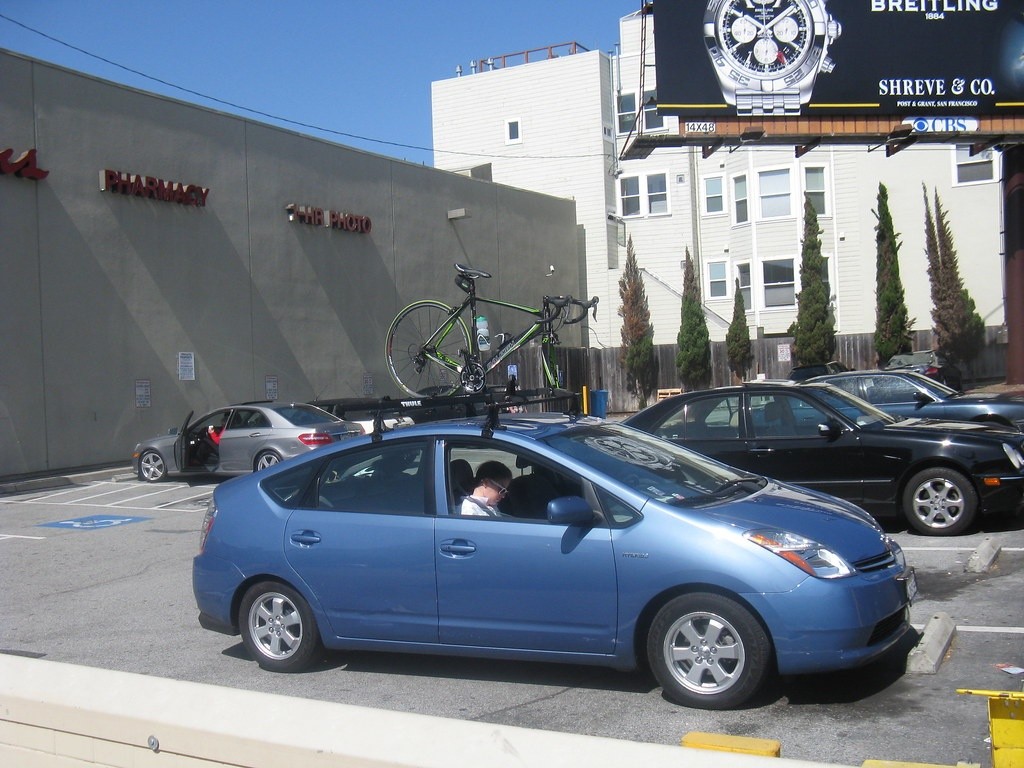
[785,361,856,410]
[733,368,1024,438]
[131,400,366,484]
[882,350,964,393]
[192,387,930,712]
[614,377,1024,536]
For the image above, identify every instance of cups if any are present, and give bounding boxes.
[209,425,214,432]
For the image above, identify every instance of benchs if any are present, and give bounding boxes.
[327,472,412,511]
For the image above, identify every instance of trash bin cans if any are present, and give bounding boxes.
[591,390,608,420]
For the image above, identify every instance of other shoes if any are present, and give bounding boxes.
[190,459,201,465]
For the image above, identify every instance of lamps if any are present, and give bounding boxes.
[839,232,844,238]
[720,160,724,166]
[723,244,729,251]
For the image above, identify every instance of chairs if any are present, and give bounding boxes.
[450,459,474,506]
[728,410,739,438]
[298,415,312,424]
[764,402,781,436]
[224,412,242,429]
[505,455,545,519]
[866,387,882,404]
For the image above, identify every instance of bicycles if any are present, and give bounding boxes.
[382,263,600,407]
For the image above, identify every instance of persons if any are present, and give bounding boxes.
[509,385,527,413]
[457,461,512,517]
[506,375,516,396]
[191,413,230,464]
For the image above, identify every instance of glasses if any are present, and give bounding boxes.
[481,479,510,496]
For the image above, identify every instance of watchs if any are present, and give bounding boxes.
[703,0,841,116]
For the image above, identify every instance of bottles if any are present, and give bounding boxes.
[476,316,491,351]
